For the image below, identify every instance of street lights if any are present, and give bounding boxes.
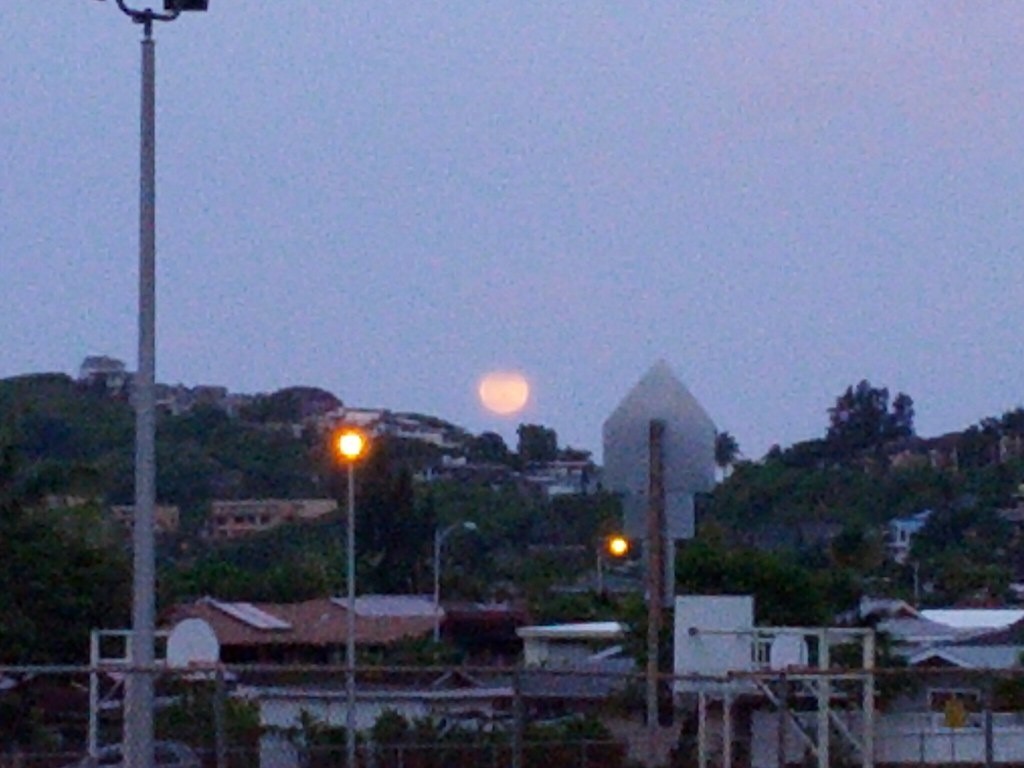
[433,520,476,664]
[338,431,364,768]
[597,536,626,598]
[101,0,207,768]
